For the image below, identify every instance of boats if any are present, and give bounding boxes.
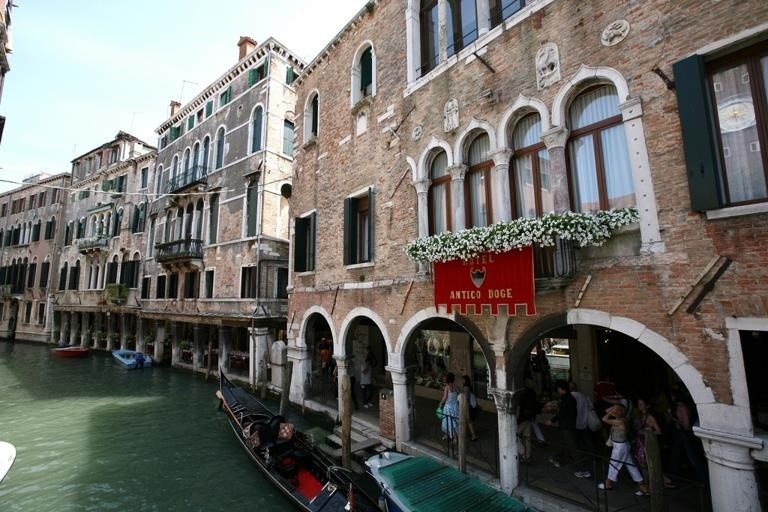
[216,365,384,512]
[364,450,542,512]
[50,346,89,358]
[112,349,152,370]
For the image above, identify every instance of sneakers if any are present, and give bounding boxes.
[636,491,650,496]
[599,483,612,490]
[549,457,561,468]
[573,471,590,478]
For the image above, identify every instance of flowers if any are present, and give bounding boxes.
[404,200,641,267]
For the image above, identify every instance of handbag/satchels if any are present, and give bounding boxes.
[436,408,444,420]
[589,412,600,431]
[605,438,612,447]
[469,394,476,409]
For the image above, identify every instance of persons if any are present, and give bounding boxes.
[318,336,378,410]
[462,374,478,441]
[522,349,551,395]
[405,335,449,374]
[513,375,705,497]
[438,372,460,445]
